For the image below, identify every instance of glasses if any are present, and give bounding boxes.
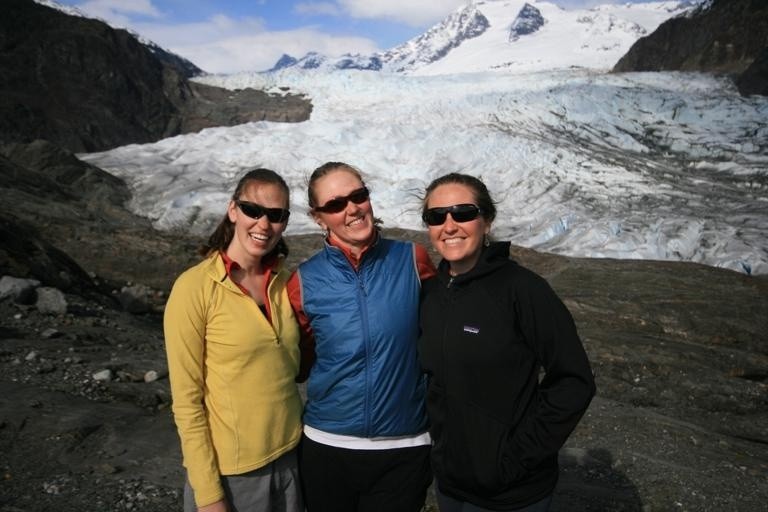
[234,200,289,222]
[421,204,479,225]
[315,188,368,212]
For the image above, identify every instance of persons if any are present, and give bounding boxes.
[410,176,595,510]
[281,161,440,512]
[162,169,309,510]
